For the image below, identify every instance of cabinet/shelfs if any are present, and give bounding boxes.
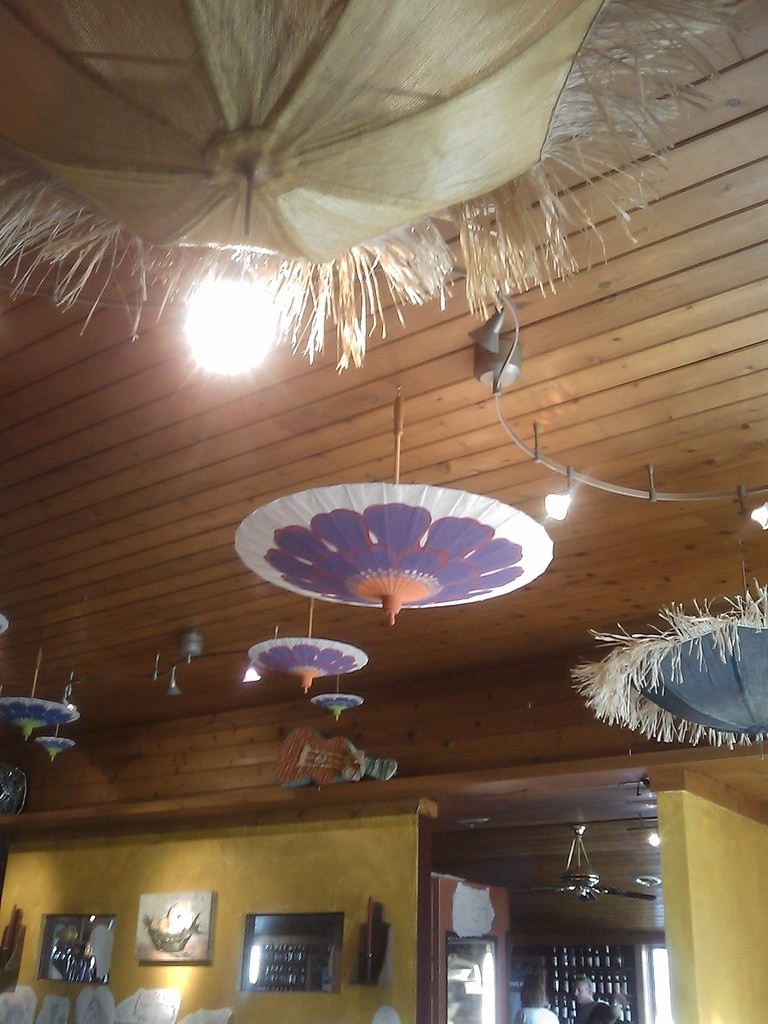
[544,945,638,1024]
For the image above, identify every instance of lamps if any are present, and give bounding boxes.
[465,302,507,354]
[165,662,181,697]
[58,671,81,710]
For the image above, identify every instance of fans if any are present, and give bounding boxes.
[511,825,656,903]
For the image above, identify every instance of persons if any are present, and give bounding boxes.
[572,976,626,1024]
[513,979,560,1024]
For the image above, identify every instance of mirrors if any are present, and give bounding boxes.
[444,930,499,1024]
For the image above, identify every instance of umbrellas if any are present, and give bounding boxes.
[311,675,364,722]
[235,388,554,626]
[35,723,76,761]
[249,596,368,694]
[0,0,749,375]
[570,557,768,760]
[0,648,81,742]
